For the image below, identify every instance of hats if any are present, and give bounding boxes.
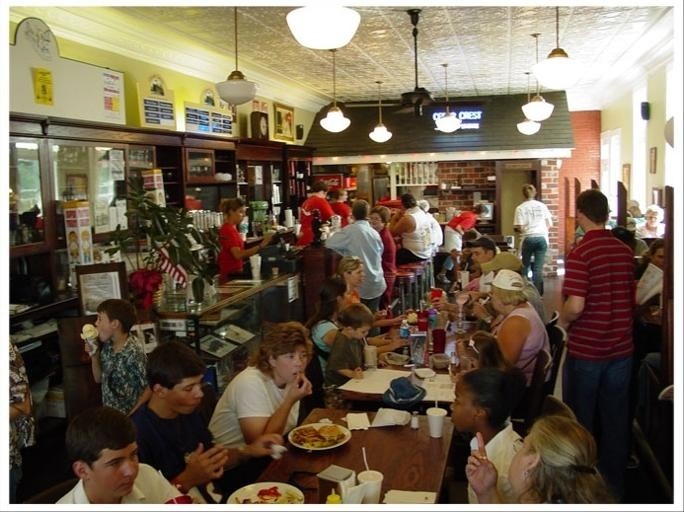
[484,269,525,290]
[479,251,524,273]
[462,237,496,250]
[382,376,427,409]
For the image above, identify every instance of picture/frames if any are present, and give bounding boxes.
[652,188,662,206]
[622,164,631,202]
[650,146,657,174]
[273,102,295,142]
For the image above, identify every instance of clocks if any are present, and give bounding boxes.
[250,111,269,140]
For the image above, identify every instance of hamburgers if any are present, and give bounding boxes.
[319,425,345,443]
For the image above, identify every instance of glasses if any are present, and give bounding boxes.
[513,438,535,452]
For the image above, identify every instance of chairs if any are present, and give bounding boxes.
[531,325,568,401]
[544,307,561,331]
[514,351,550,432]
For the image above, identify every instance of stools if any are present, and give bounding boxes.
[415,258,433,295]
[394,271,414,316]
[398,263,426,310]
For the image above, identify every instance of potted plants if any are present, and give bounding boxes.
[99,164,225,312]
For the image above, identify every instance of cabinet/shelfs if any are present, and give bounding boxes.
[123,124,158,234]
[10,292,83,441]
[181,131,238,227]
[9,119,58,297]
[128,272,305,418]
[235,136,286,232]
[40,121,131,247]
[284,145,317,218]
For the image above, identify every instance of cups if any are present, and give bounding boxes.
[363,346,377,368]
[249,256,262,279]
[357,469,385,505]
[417,311,428,331]
[426,408,447,439]
[460,271,469,289]
[431,288,442,302]
[432,329,446,353]
[187,210,224,228]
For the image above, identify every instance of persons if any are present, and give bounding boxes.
[9,177,666,503]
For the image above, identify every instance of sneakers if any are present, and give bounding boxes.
[435,273,451,284]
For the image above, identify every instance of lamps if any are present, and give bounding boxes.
[318,50,352,134]
[285,6,362,51]
[516,72,542,136]
[368,81,393,143]
[521,32,555,122]
[433,64,462,134]
[215,7,258,108]
[531,7,583,91]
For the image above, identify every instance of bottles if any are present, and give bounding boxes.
[324,487,343,504]
[397,320,411,353]
[408,411,420,429]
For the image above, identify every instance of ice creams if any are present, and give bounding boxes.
[80,323,99,357]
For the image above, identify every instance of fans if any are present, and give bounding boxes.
[395,9,486,116]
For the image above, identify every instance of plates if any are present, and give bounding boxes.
[284,422,356,451]
[415,368,436,379]
[223,481,306,504]
